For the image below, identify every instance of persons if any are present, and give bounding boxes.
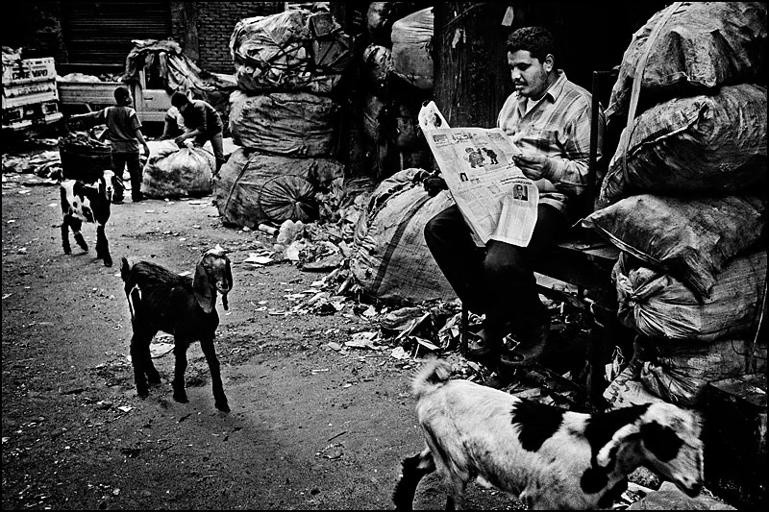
[106,87,149,204]
[463,147,498,168]
[460,173,468,181]
[424,26,605,367]
[514,185,527,200]
[160,92,226,173]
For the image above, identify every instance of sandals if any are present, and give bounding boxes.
[462,311,550,366]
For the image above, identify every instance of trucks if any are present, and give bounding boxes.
[0,39,238,129]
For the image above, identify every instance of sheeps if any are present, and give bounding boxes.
[59,170,125,267]
[120,242,233,414]
[388,360,702,512]
